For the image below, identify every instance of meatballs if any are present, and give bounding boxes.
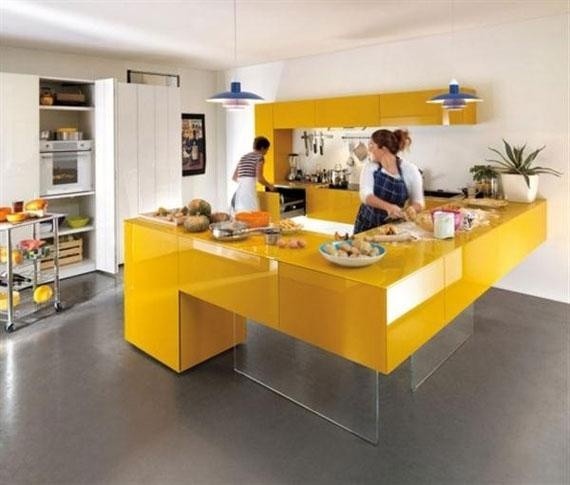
[322,239,378,259]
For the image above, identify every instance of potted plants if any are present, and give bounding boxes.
[485,137,566,204]
[467,165,499,195]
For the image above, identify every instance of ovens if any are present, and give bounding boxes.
[39,140,94,196]
[266,186,306,216]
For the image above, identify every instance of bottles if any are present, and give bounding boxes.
[12,201,24,213]
[191,130,202,164]
[304,169,326,183]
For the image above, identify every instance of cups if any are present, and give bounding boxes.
[265,229,279,245]
[467,181,482,199]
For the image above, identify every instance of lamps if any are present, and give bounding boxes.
[204,0,266,113]
[424,0,485,113]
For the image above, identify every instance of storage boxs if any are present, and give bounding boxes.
[40,235,83,270]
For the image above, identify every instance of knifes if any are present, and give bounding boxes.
[312,131,318,153]
[319,133,324,155]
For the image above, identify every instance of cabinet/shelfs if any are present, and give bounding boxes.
[37,75,116,286]
[0,72,36,291]
[113,82,182,265]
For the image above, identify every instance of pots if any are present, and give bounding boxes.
[328,170,349,184]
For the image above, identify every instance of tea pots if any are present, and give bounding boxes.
[39,85,54,105]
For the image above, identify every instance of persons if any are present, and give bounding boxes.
[354,128,425,235]
[232,136,275,212]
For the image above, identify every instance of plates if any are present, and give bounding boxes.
[318,240,384,266]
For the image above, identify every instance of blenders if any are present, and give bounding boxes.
[287,153,298,180]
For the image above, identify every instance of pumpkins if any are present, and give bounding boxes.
[188,200,210,215]
[184,212,209,232]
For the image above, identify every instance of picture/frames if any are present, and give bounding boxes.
[127,69,181,88]
[181,113,207,176]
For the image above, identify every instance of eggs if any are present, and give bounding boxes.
[276,238,306,249]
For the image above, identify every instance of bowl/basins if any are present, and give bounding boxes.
[67,214,91,228]
[208,221,250,240]
[57,131,84,142]
[6,212,26,222]
[0,291,20,309]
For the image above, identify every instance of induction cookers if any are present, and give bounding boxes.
[327,184,348,189]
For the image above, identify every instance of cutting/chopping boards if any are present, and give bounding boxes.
[141,208,179,226]
